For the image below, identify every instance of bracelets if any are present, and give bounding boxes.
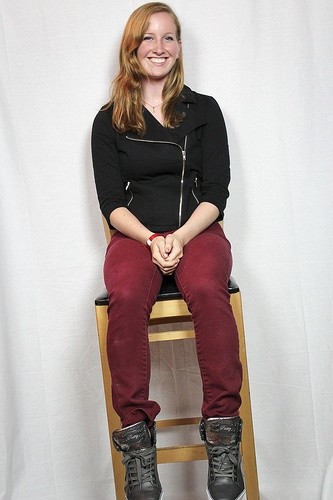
[146,233,166,248]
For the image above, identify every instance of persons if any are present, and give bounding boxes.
[91,2,246,500]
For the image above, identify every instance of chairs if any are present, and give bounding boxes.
[94,214,260,500]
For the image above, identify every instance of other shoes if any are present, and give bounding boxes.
[198,416,246,500]
[111,421,164,500]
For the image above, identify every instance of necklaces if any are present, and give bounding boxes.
[143,100,163,113]
